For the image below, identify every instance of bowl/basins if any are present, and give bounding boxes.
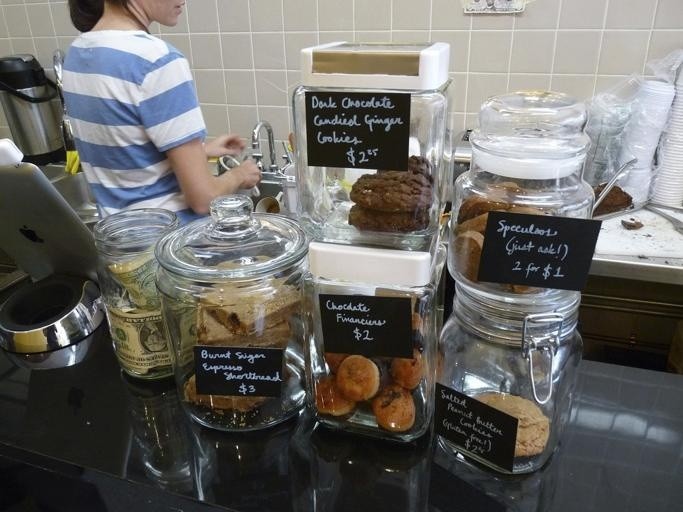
[216,155,259,199]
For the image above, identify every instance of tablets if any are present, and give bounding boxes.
[0,162,102,285]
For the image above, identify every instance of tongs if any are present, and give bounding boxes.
[589,157,647,221]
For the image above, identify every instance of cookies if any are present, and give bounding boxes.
[473,393,551,458]
[316,288,426,431]
[348,155,434,232]
[183,256,305,413]
[591,183,632,217]
[450,183,549,295]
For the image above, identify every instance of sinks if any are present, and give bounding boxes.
[251,174,282,212]
[49,161,100,215]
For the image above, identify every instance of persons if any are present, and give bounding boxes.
[143,323,167,352]
[62,0,261,227]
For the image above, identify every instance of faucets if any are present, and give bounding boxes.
[252,120,278,173]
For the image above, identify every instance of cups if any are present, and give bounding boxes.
[89,207,184,385]
[0,54,70,164]
[603,60,682,207]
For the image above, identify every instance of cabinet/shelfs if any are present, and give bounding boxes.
[575,274,683,374]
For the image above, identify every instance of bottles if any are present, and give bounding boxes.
[115,366,193,485]
[151,193,309,436]
[291,39,452,251]
[429,283,577,470]
[298,239,437,442]
[449,91,593,300]
[301,431,430,511]
[184,423,316,511]
